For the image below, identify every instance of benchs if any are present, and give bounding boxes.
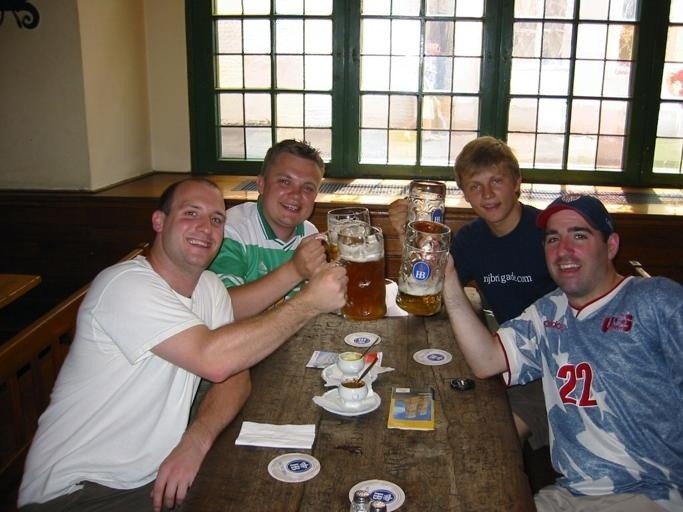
[1,241,156,509]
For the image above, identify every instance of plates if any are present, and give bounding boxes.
[320,363,378,387]
[318,388,381,416]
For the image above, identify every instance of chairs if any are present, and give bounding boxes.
[621,256,653,284]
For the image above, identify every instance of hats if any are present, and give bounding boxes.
[535,193,615,232]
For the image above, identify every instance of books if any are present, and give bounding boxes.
[386,386,435,432]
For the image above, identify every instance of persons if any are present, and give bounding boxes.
[194,139,327,408]
[15,175,348,512]
[388,136,548,450]
[411,194,680,511]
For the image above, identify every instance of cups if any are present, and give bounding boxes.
[393,179,449,317]
[337,378,368,406]
[337,352,365,378]
[309,207,389,320]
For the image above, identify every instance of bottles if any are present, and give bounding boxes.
[349,491,388,511]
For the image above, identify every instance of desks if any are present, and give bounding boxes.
[1,262,39,312]
